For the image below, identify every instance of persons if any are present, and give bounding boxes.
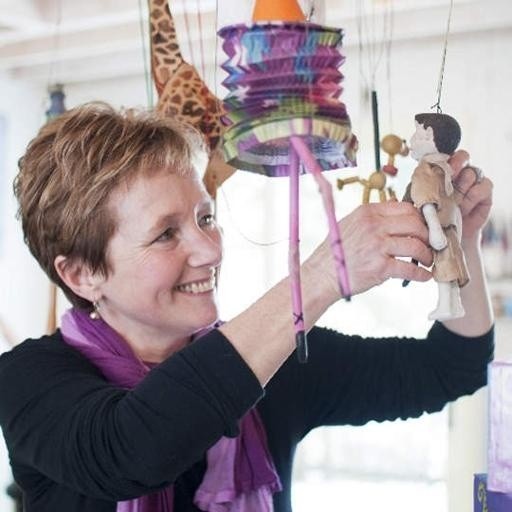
[0,100,494,512]
[410,113,470,287]
